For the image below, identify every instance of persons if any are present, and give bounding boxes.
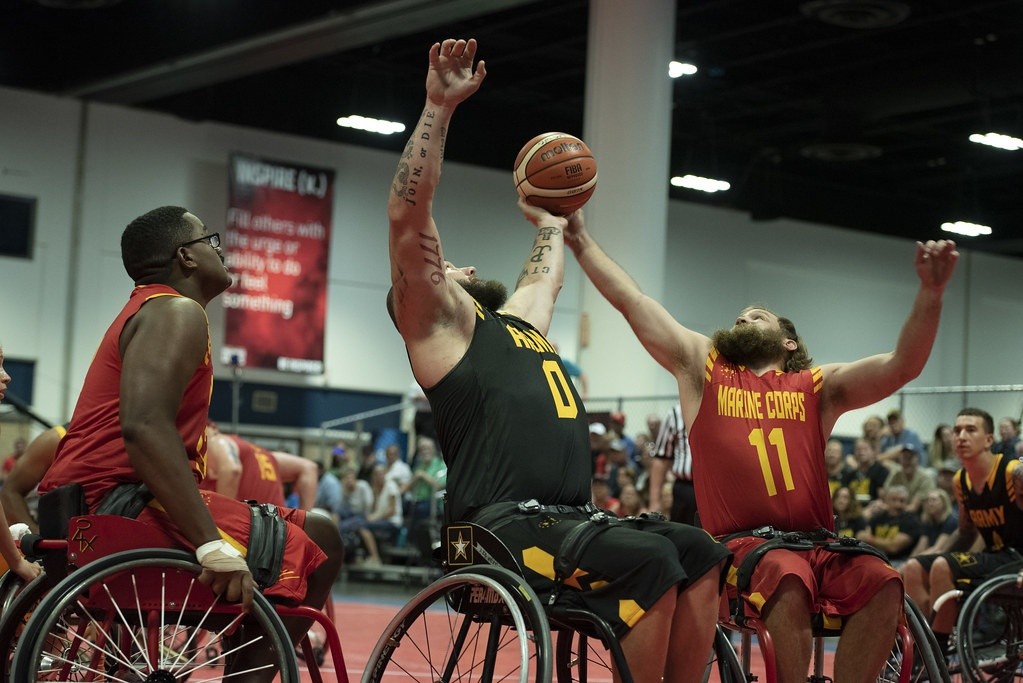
[648,405,698,526]
[284,431,448,570]
[880,407,1023,683]
[587,412,676,521]
[387,37,736,683]
[0,206,343,683]
[562,206,959,683]
[826,411,1023,578]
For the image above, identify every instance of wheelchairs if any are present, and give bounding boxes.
[670,479,954,683]
[901,558,1023,683]
[346,496,749,683]
[1,483,355,683]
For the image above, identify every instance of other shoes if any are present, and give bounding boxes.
[364,558,382,569]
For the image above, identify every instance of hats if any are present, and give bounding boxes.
[940,466,955,476]
[612,413,624,424]
[887,408,901,419]
[901,443,919,453]
[589,422,605,435]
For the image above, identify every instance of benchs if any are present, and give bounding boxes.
[331,540,445,587]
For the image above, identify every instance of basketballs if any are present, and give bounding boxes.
[512,130,600,214]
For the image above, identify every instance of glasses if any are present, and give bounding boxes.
[172,233,221,259]
